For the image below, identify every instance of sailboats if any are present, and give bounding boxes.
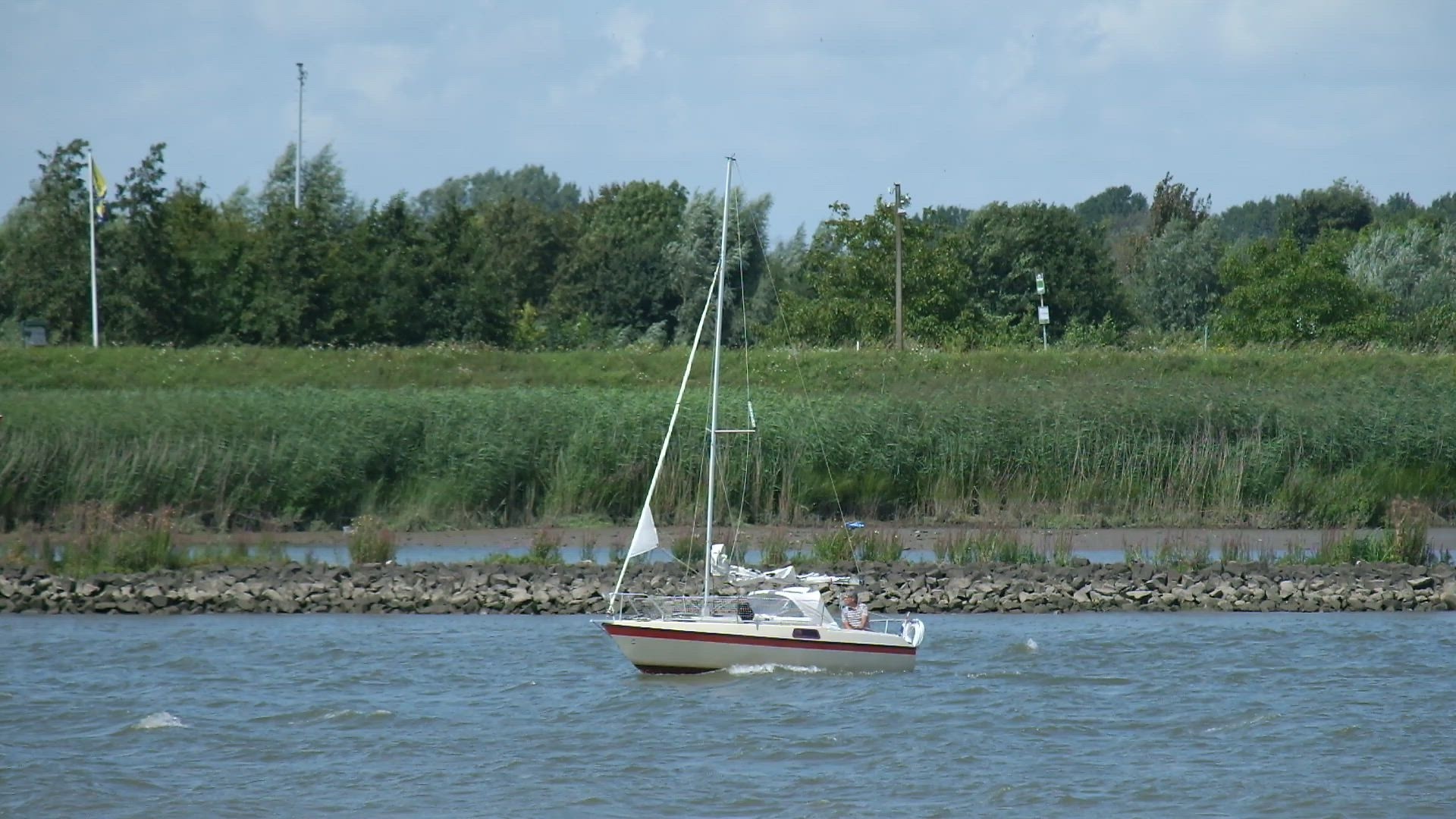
[588,151,925,675]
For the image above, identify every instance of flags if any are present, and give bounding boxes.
[92,156,110,226]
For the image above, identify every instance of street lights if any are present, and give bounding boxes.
[294,61,307,226]
[884,180,913,350]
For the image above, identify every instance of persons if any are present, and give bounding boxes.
[841,592,868,631]
